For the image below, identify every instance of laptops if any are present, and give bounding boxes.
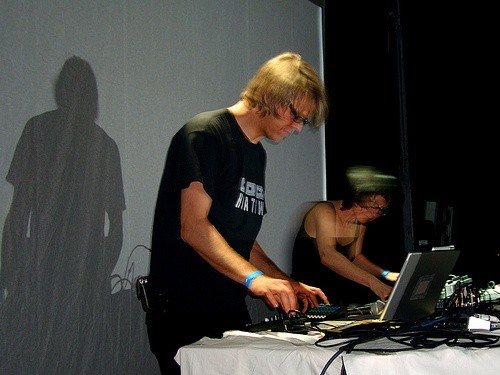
[333,250,461,321]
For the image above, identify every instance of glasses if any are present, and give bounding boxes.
[367,199,387,216]
[287,100,312,125]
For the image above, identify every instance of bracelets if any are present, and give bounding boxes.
[381,270,389,277]
[245,271,264,288]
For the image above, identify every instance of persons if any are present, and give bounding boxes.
[146,52,330,374]
[290,184,401,304]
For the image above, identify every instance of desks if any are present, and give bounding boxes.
[174,329,500,375]
